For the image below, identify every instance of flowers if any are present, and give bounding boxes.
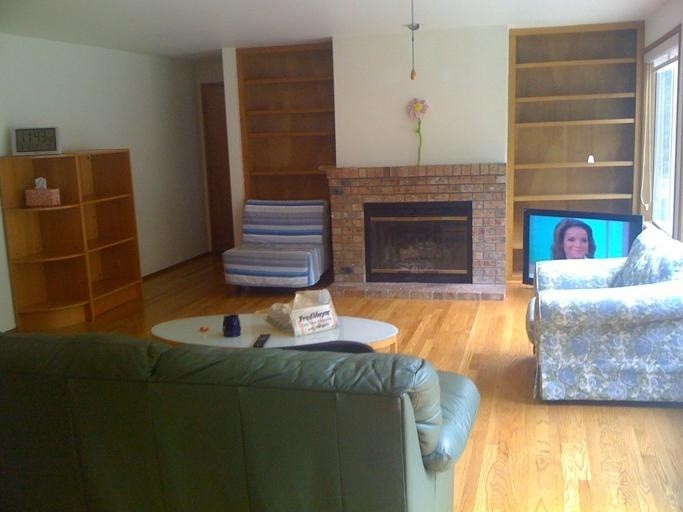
[405,98,432,166]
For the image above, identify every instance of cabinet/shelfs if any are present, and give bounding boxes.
[7,148,145,328]
[507,18,646,288]
[233,36,336,199]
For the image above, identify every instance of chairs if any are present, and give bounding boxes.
[527,220,682,411]
[227,198,332,295]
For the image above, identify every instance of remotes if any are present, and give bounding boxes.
[254,335,269,347]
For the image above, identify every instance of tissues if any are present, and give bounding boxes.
[25,177,61,207]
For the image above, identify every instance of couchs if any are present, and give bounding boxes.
[0,330,482,512]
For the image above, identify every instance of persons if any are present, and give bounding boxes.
[550,217,596,260]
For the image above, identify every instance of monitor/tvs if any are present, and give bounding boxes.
[523,208,642,286]
